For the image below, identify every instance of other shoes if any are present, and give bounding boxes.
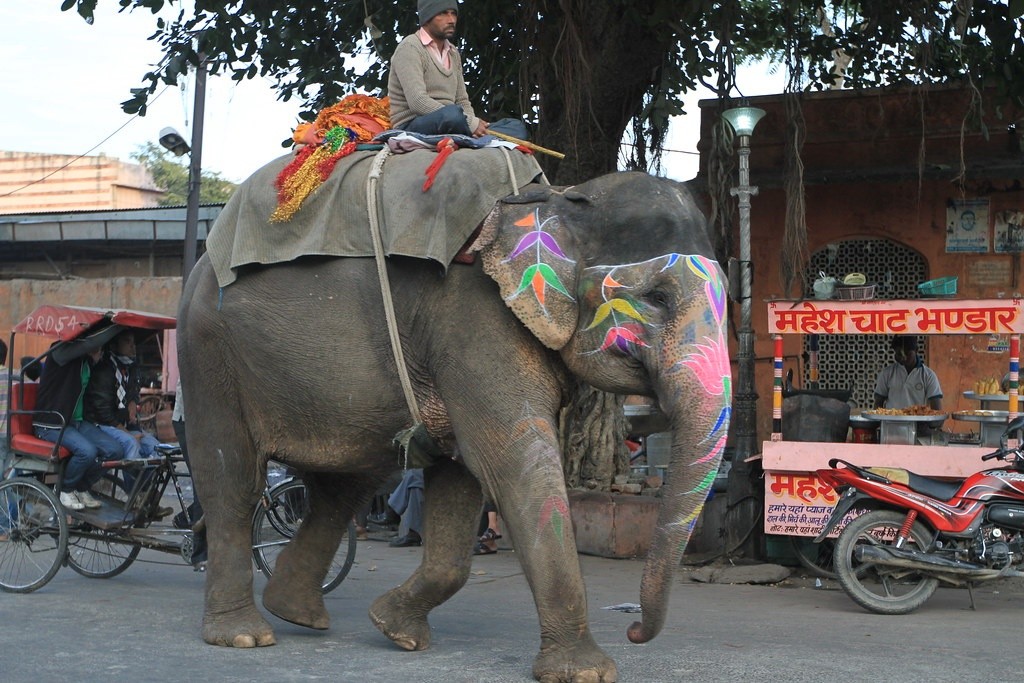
[367,512,401,526]
[389,535,421,548]
[172,504,193,529]
[138,501,173,517]
[342,528,368,540]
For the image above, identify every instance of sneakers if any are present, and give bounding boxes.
[74,490,102,507]
[53,485,84,509]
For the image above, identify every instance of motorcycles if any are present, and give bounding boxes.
[813,416,1024,614]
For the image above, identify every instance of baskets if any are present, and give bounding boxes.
[917,275,958,296]
[835,285,877,302]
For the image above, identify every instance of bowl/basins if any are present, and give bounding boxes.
[848,415,880,429]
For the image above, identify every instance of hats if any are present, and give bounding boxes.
[417,0,459,27]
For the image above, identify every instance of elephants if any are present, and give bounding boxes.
[175,145,735,683]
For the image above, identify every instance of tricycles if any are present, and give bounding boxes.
[0,301,358,593]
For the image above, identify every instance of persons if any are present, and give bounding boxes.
[387,0,529,141]
[872,335,944,435]
[353,444,503,554]
[0,331,208,571]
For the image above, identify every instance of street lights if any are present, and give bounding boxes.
[721,99,767,566]
[158,125,202,297]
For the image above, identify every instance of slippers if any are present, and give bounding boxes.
[473,544,499,556]
[479,528,503,542]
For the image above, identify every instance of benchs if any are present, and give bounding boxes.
[10,383,72,459]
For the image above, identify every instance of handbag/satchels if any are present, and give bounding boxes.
[813,271,838,301]
